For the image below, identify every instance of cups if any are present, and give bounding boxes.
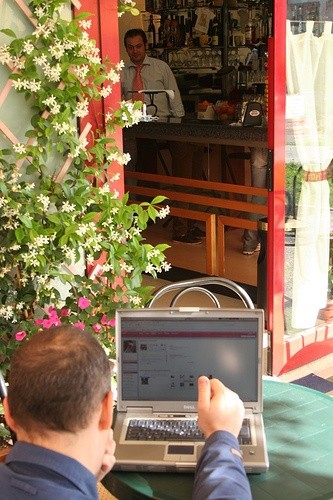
[199,35,212,47]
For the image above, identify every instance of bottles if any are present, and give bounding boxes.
[143,43,223,71]
[140,0,197,46]
[211,18,222,47]
[225,0,274,127]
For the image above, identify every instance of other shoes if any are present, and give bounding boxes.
[243,242,261,254]
[172,233,202,245]
[192,227,205,239]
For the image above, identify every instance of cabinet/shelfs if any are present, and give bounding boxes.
[144,0,274,96]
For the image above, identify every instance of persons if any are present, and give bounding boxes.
[243,147,272,255]
[0,326,253,500]
[119,29,203,245]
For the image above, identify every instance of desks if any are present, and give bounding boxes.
[124,115,266,305]
[101,377,333,500]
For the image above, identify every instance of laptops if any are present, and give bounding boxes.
[110,305,270,474]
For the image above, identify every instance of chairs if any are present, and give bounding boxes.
[145,277,256,309]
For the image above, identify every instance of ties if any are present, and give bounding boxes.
[132,65,144,102]
